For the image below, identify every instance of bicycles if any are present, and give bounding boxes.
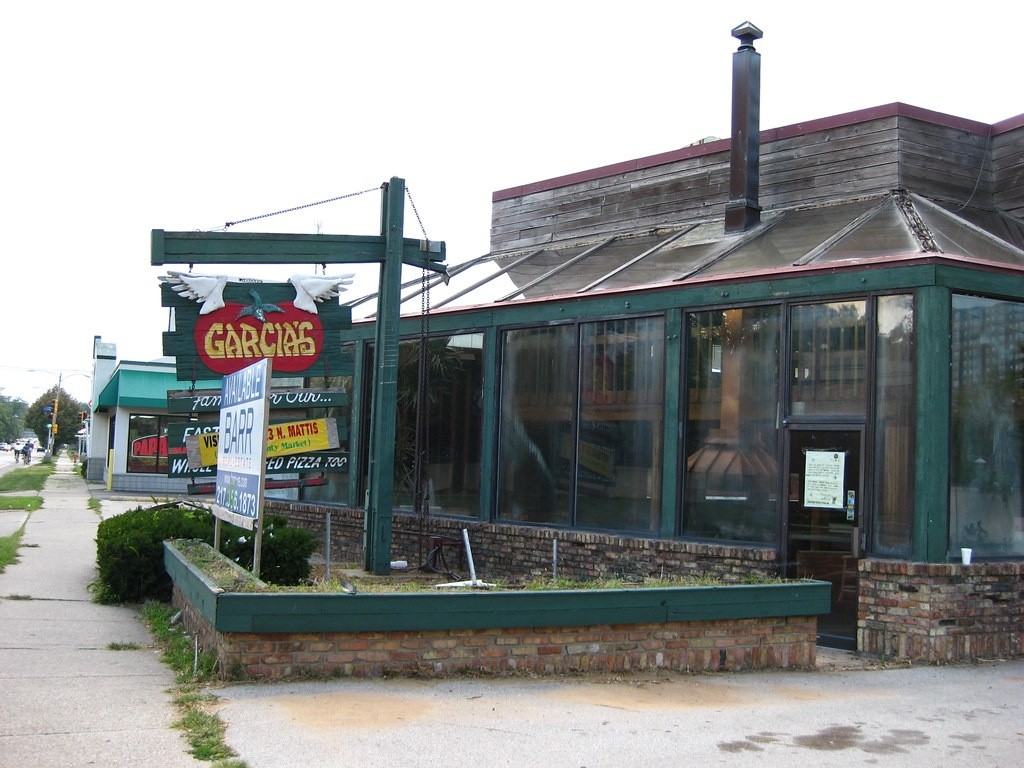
[15,452,31,466]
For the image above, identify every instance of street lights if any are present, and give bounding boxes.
[27,368,92,455]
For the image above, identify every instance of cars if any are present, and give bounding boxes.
[0,438,45,452]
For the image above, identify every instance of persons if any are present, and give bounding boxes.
[14,439,34,460]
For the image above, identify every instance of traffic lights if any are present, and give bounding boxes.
[47,424,52,428]
[51,399,57,414]
[78,411,83,425]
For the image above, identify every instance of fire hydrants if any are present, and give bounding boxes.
[73,453,78,463]
